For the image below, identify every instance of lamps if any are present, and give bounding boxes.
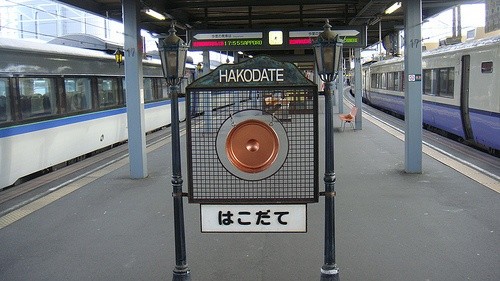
[113,48,124,69]
[197,62,203,73]
[154,23,191,87]
[312,19,346,82]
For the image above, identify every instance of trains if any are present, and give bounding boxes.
[347,35,500,155]
[0,31,279,190]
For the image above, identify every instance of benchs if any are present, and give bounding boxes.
[339,106,357,133]
